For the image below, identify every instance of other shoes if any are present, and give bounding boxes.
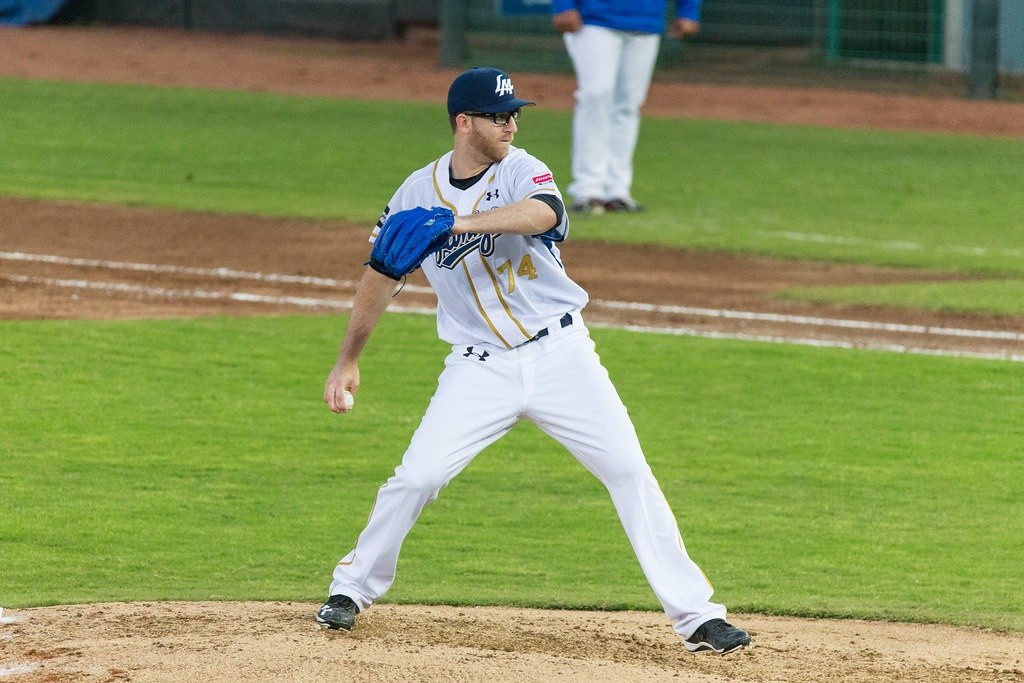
[606,198,643,212]
[573,202,605,215]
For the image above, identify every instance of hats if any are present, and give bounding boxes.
[447,66,536,112]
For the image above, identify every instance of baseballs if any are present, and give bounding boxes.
[336,391,355,411]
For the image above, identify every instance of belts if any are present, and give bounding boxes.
[521,313,571,341]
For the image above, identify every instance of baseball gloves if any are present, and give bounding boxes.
[361,204,455,298]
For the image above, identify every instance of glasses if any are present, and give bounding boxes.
[466,108,520,125]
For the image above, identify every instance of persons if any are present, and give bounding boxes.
[551,0,703,215]
[317,67,752,657]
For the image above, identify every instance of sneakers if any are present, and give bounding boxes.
[685,618,750,656]
[318,594,359,631]
